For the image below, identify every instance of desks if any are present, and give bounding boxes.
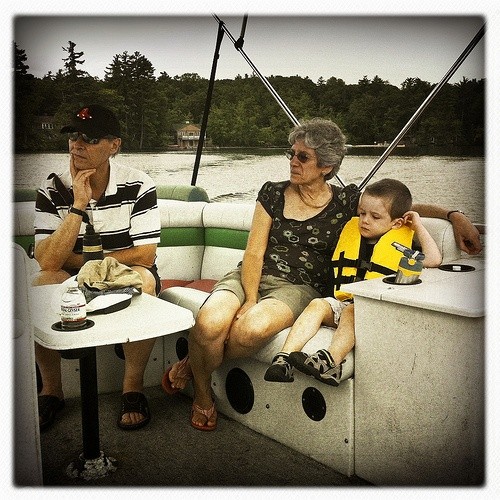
[28,283,196,480]
[338,260,485,487]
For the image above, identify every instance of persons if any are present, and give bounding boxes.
[29,106,160,430]
[161,119,483,430]
[264,179,442,386]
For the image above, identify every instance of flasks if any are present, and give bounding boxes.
[395,250,425,285]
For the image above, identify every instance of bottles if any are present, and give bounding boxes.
[59,281,87,331]
[82,225,104,263]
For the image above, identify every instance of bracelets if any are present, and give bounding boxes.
[68,205,87,217]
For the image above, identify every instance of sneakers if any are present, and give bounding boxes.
[290,349,346,387]
[264,352,295,382]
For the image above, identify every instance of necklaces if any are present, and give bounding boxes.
[298,184,332,208]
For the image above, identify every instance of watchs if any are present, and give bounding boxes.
[447,209,464,220]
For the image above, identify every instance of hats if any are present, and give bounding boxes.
[61,105,120,139]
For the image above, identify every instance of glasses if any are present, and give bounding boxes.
[67,129,115,144]
[284,149,319,163]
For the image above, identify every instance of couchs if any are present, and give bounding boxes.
[14,184,459,485]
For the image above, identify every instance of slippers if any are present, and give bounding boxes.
[119,392,150,430]
[38,395,71,432]
[191,401,217,430]
[162,366,185,394]
